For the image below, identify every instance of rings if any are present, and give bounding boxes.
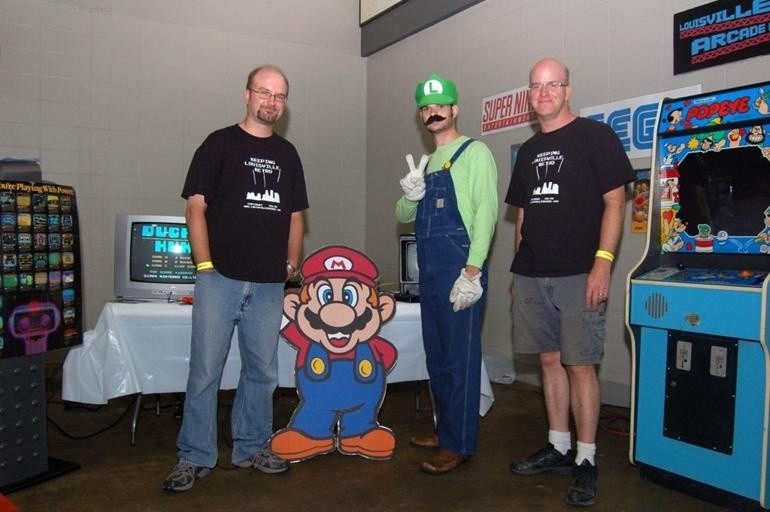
[598,291,602,297]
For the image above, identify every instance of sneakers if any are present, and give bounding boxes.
[247,446,287,473]
[567,438,600,506]
[408,431,441,449]
[162,459,203,491]
[422,447,466,473]
[510,441,578,475]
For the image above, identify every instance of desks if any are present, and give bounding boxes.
[60,300,495,447]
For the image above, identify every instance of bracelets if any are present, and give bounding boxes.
[594,250,616,264]
[196,261,214,270]
[286,259,297,273]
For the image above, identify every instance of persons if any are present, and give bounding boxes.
[503,59,637,507]
[162,66,310,492]
[395,75,500,475]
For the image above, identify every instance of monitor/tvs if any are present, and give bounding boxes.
[114,212,201,300]
[399,232,423,297]
[674,145,768,239]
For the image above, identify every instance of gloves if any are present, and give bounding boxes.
[449,267,485,313]
[400,154,430,202]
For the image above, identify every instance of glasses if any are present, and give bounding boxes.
[525,81,569,91]
[249,88,290,104]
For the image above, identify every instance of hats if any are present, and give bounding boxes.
[415,74,458,110]
